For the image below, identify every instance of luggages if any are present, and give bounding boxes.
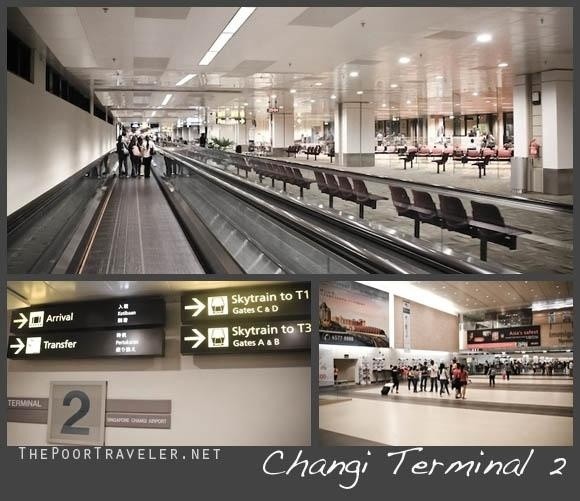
[381,386,390,395]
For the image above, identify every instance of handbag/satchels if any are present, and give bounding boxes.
[408,376,411,379]
[132,146,142,157]
[150,148,156,155]
[122,146,129,155]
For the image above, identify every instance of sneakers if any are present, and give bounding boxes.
[119,174,150,178]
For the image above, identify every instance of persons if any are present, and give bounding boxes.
[480,130,495,150]
[110,128,157,178]
[326,131,334,141]
[199,133,205,147]
[390,358,574,399]
[467,130,476,137]
[334,364,339,384]
[376,130,384,146]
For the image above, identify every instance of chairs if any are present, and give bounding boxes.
[375,145,514,178]
[225,152,532,262]
[286,145,335,163]
